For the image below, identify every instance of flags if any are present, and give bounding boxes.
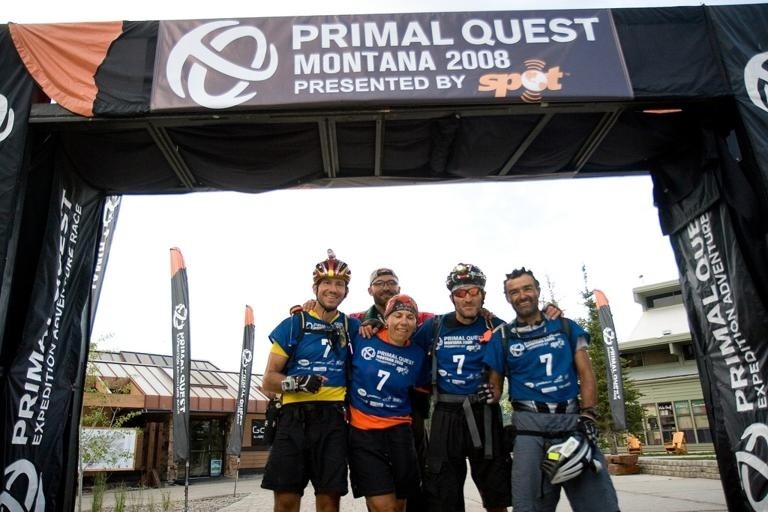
[593,290,625,429]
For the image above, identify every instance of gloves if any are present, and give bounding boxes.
[475,381,498,409]
[280,374,328,394]
[576,408,600,445]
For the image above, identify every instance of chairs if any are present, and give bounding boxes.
[626,436,642,455]
[665,431,688,454]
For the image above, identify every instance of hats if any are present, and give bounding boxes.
[369,268,399,286]
[383,294,418,319]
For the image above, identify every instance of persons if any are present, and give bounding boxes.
[303,267,496,511]
[360,263,563,511]
[346,293,432,511]
[259,260,361,511]
[476,267,621,511]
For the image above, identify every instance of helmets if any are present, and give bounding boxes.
[538,431,603,500]
[446,263,487,290]
[312,248,352,284]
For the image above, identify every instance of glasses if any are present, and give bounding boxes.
[371,280,398,286]
[452,287,482,298]
